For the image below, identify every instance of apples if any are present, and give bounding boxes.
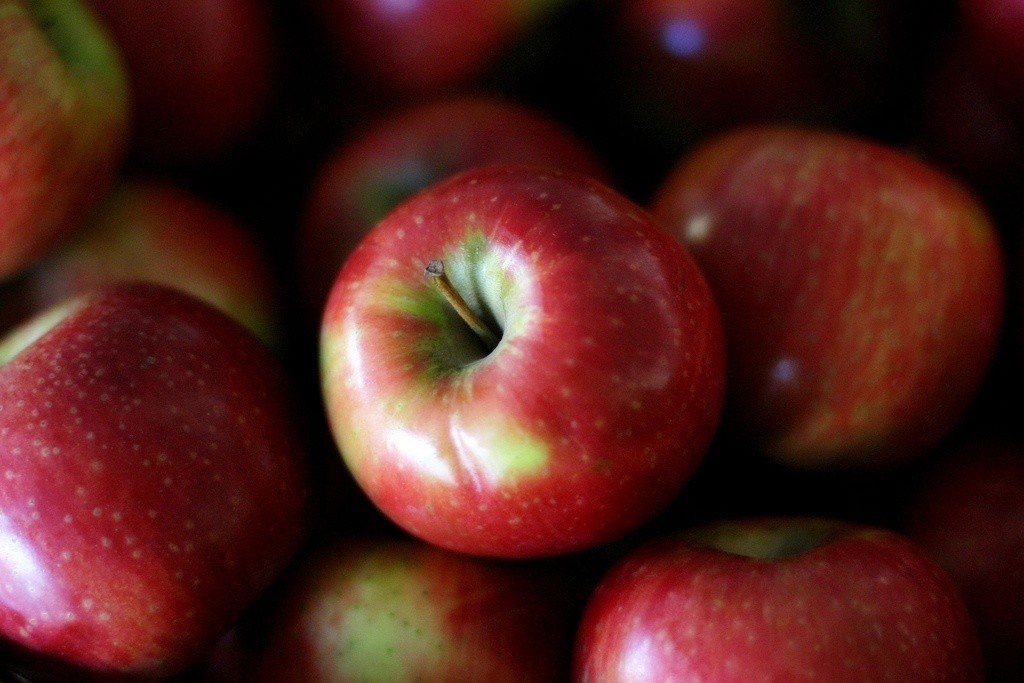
[0,0,1024,683]
